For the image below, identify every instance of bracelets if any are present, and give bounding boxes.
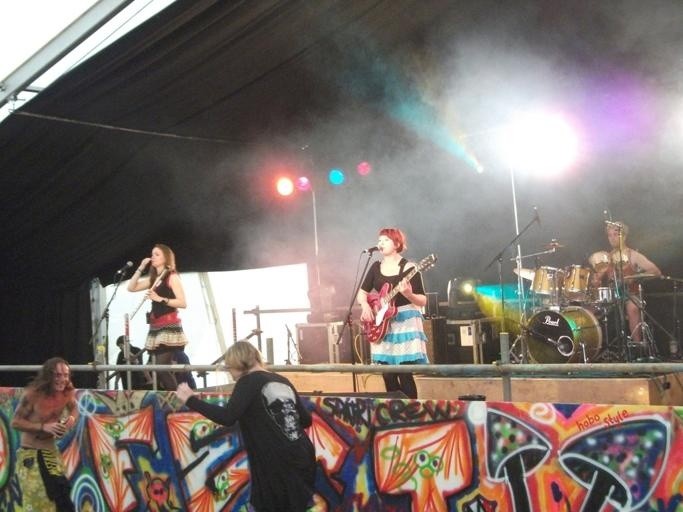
[40,423,44,431]
[162,297,168,304]
[69,416,75,422]
[137,268,143,274]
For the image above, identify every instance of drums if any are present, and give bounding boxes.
[610,248,631,269]
[564,264,594,303]
[597,287,619,304]
[526,306,602,364]
[590,251,611,274]
[534,265,564,296]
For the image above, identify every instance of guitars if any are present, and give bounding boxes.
[359,253,436,343]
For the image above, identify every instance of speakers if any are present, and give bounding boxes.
[296,321,364,364]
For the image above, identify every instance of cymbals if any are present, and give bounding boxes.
[624,273,654,279]
[512,268,535,280]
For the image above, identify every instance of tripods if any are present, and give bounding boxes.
[483,217,683,363]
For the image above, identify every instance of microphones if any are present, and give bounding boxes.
[117,261,134,274]
[295,345,303,361]
[363,245,382,255]
[533,204,542,227]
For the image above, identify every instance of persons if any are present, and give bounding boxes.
[175,341,316,512]
[357,228,431,399]
[592,222,662,341]
[9,357,80,512]
[114,335,146,389]
[129,243,197,391]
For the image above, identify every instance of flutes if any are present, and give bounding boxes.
[129,265,169,321]
